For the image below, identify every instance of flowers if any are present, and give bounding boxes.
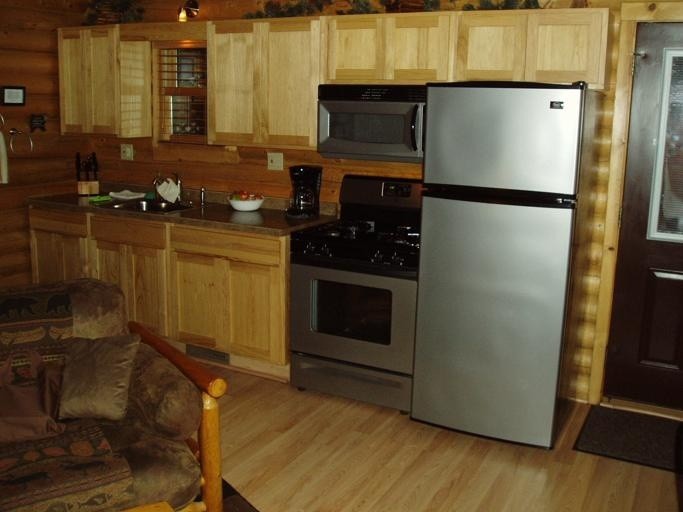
[55,334,145,417]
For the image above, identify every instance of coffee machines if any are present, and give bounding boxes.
[286,164,322,220]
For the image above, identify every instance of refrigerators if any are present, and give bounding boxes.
[407,82,600,447]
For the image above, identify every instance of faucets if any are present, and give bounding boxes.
[153,177,183,202]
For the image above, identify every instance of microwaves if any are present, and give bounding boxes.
[314,85,427,165]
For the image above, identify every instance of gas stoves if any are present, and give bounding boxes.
[289,217,421,269]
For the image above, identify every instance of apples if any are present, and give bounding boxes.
[228,190,263,201]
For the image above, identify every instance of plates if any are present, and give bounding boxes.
[226,196,263,212]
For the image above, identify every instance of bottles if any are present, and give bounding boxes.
[200,187,205,205]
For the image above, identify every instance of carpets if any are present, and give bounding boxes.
[572,405,683,471]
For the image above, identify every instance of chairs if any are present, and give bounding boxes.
[0,278,226,511]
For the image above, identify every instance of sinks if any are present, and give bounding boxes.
[96,196,195,216]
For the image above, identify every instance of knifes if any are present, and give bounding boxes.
[75,151,98,180]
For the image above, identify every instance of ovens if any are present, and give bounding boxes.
[288,252,417,416]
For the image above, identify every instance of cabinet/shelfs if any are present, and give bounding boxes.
[211,17,319,148]
[91,214,167,347]
[453,9,609,83]
[323,12,450,82]
[28,209,89,287]
[59,23,120,135]
[168,221,290,381]
